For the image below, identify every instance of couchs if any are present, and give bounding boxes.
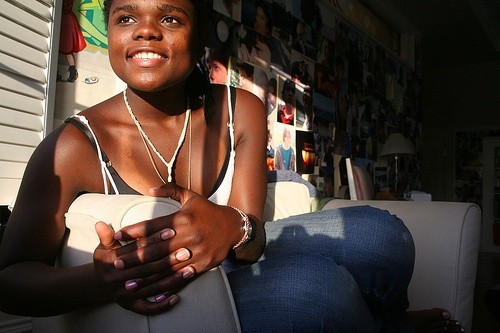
[32,170,482,333]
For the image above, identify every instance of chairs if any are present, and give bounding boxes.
[345,158,375,200]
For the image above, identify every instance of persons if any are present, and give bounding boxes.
[221,0,485,190]
[0,0,465,333]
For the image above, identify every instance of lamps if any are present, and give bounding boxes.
[380,133,416,191]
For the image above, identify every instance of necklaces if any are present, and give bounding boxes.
[123,85,195,196]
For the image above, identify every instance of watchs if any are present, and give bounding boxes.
[224,204,252,254]
